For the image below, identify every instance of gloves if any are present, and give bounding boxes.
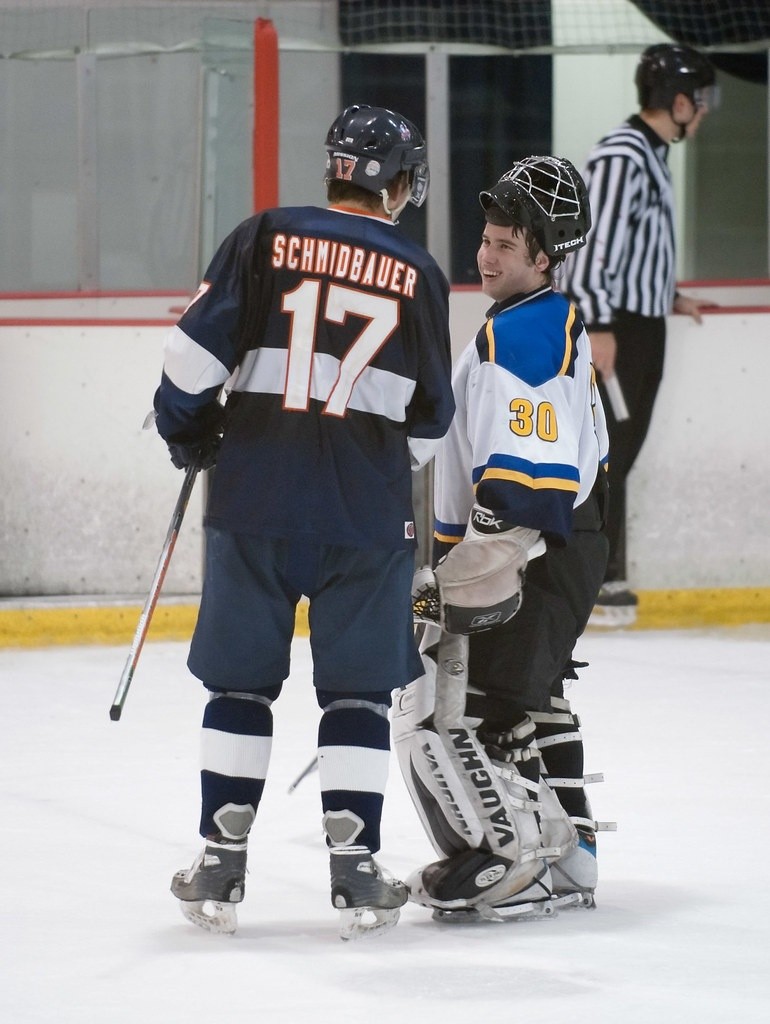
[170,419,223,469]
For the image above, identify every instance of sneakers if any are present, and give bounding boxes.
[170,834,248,934]
[583,580,638,627]
[330,846,410,942]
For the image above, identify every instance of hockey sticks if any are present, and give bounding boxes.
[282,564,441,799]
[107,390,222,722]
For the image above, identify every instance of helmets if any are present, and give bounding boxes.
[479,155,591,254]
[324,104,427,196]
[634,44,715,90]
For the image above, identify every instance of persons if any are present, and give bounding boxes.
[412,155,614,926]
[150,101,456,911]
[564,44,722,617]
